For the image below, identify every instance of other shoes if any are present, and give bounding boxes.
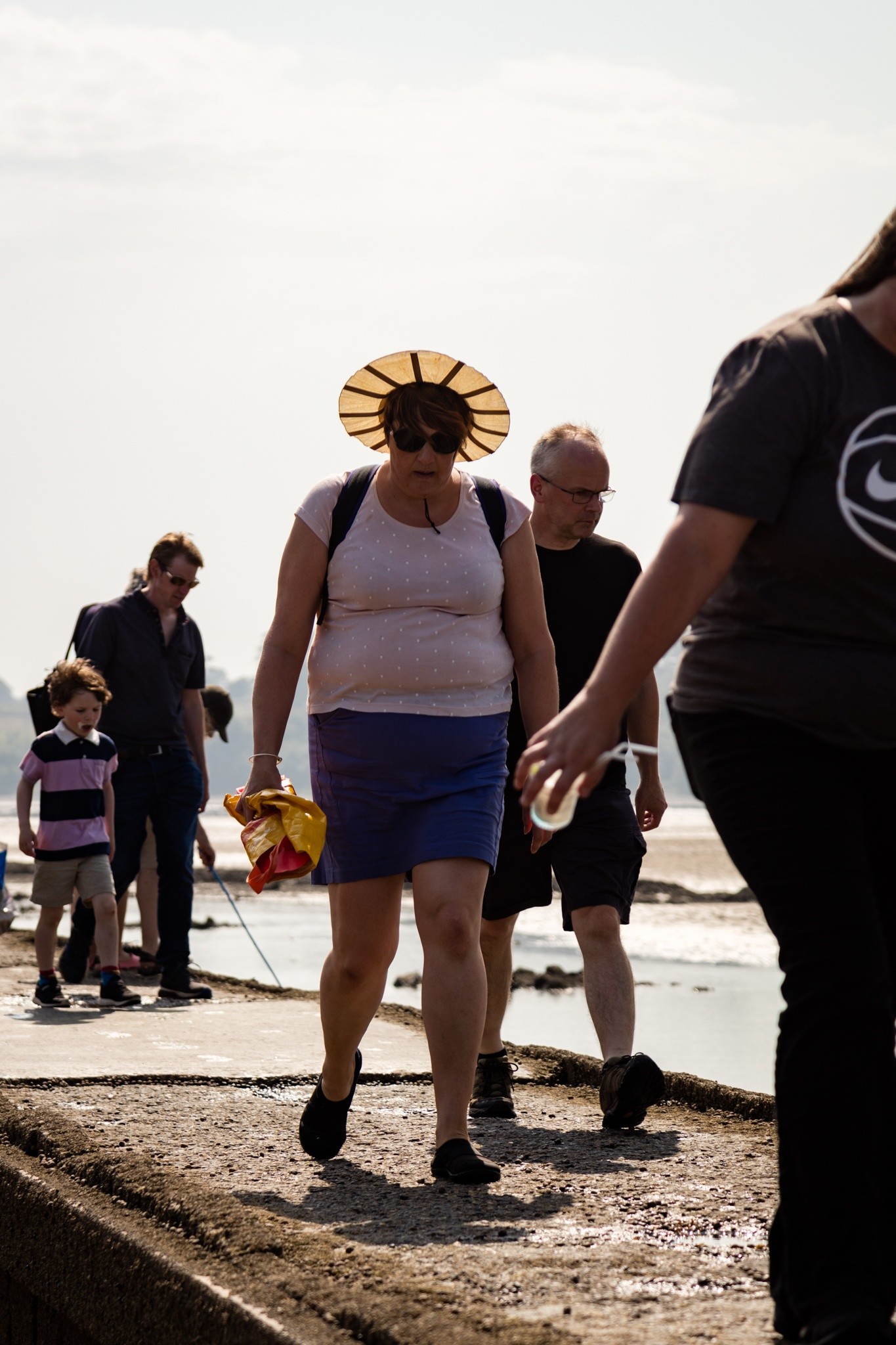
[300,1048,363,1158]
[430,1139,500,1182]
[93,962,102,978]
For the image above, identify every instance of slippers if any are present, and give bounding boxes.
[118,952,142,968]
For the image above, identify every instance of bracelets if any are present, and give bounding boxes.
[248,753,283,764]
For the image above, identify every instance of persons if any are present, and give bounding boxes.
[515,202,896,1345]
[470,424,668,1129]
[16,531,232,1008]
[235,350,560,1182]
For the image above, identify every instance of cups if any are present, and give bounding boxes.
[523,760,586,831]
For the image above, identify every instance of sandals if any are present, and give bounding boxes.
[137,951,162,977]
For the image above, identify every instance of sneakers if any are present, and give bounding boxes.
[32,979,71,1007]
[469,1055,517,1117]
[98,979,141,1006]
[59,928,90,984]
[600,1054,666,1129]
[158,981,211,998]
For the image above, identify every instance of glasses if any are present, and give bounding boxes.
[538,476,617,505]
[385,421,462,455]
[163,567,199,589]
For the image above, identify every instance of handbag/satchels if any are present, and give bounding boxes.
[26,602,107,737]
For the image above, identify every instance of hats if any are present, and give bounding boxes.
[338,351,510,463]
[201,686,233,743]
